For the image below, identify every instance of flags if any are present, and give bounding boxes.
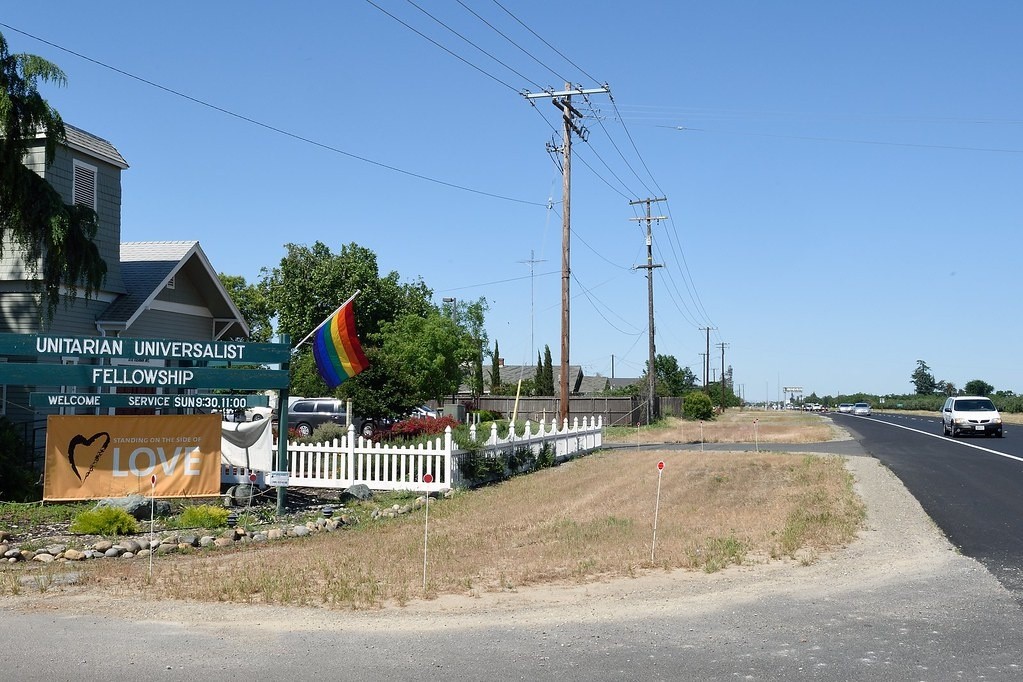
[313,300,369,389]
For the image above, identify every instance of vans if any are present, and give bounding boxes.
[941,395,1003,438]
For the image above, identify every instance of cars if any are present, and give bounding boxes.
[786,404,800,410]
[853,402,872,416]
[802,403,823,412]
[838,403,852,414]
[224,405,279,422]
[406,405,440,418]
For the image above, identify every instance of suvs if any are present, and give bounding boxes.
[288,397,388,439]
